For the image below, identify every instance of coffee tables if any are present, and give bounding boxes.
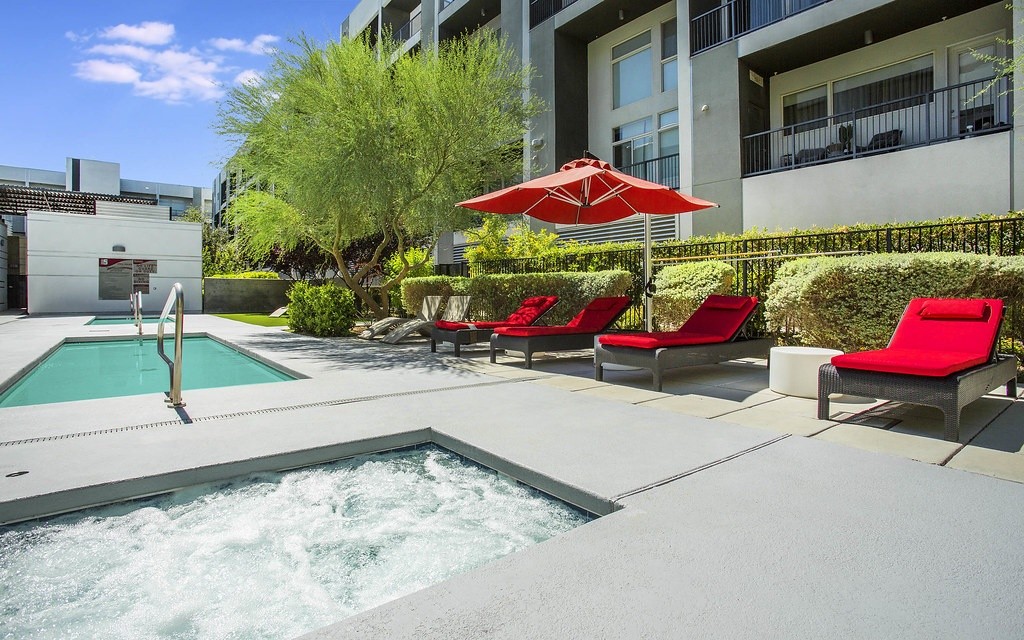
[821,141,846,159]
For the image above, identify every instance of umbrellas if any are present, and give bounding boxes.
[453,150,723,371]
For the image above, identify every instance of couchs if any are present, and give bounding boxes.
[792,146,824,167]
[857,126,901,156]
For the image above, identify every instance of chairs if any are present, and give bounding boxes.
[382,294,470,343]
[429,294,563,356]
[816,297,1020,443]
[356,295,443,340]
[595,293,774,394]
[488,295,635,370]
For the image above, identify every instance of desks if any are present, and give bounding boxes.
[769,345,844,397]
